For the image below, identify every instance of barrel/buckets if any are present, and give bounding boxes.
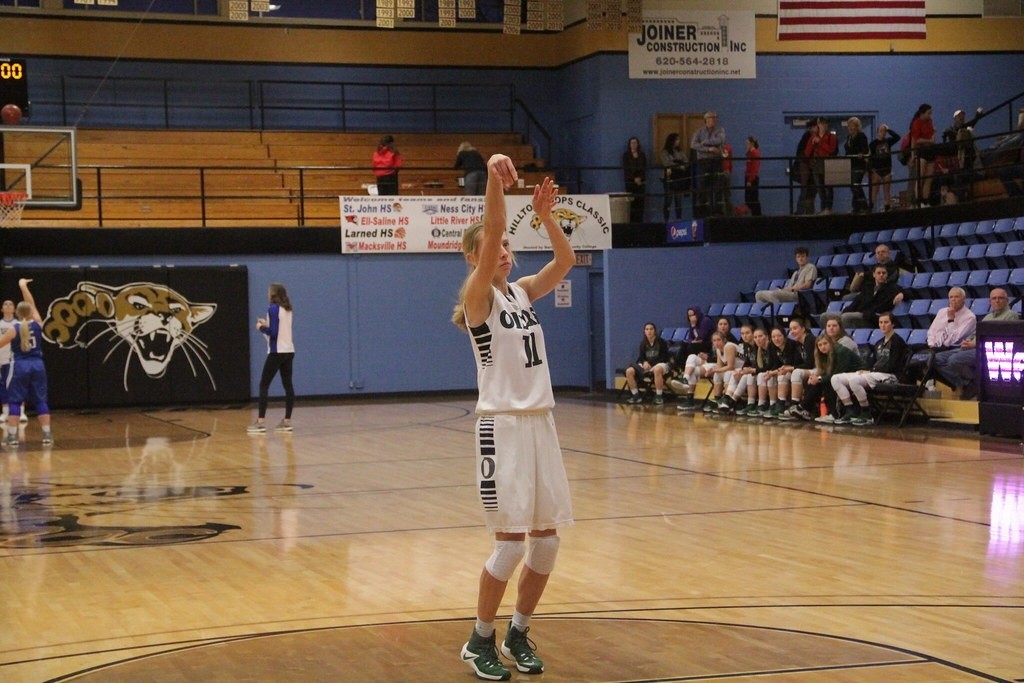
[607,191,635,225]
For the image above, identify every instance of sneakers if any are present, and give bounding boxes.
[460,626,511,680]
[626,394,642,404]
[1,434,19,446]
[42,432,52,443]
[677,396,874,426]
[655,397,663,405]
[501,620,544,673]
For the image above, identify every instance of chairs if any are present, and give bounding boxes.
[616,217,1024,430]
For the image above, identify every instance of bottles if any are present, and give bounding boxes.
[821,400,828,417]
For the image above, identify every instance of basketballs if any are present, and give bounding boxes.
[1,104,22,124]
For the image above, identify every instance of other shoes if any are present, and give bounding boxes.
[20,414,28,422]
[246,419,266,432]
[0,414,8,422]
[960,384,976,400]
[817,209,832,215]
[921,203,931,209]
[274,418,293,431]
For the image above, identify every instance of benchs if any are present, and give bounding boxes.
[0,126,568,230]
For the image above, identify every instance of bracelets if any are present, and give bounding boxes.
[948,320,954,322]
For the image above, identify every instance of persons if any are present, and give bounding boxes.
[623,137,648,223]
[791,117,901,215]
[454,142,488,196]
[820,265,904,328]
[660,132,689,223]
[910,287,976,352]
[373,135,403,196]
[941,108,983,204]
[0,279,54,445]
[755,248,817,303]
[719,142,733,217]
[452,154,575,681]
[246,284,295,432]
[691,111,725,205]
[909,104,936,208]
[842,244,894,301]
[745,137,762,216]
[935,288,1019,400]
[625,305,911,426]
